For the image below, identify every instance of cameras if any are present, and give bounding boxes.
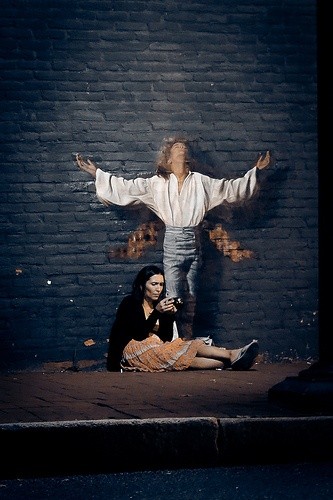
[166,296,183,309]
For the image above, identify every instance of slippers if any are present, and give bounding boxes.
[228,338,260,369]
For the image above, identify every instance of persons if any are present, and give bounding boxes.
[75,139,271,341]
[103,265,259,375]
